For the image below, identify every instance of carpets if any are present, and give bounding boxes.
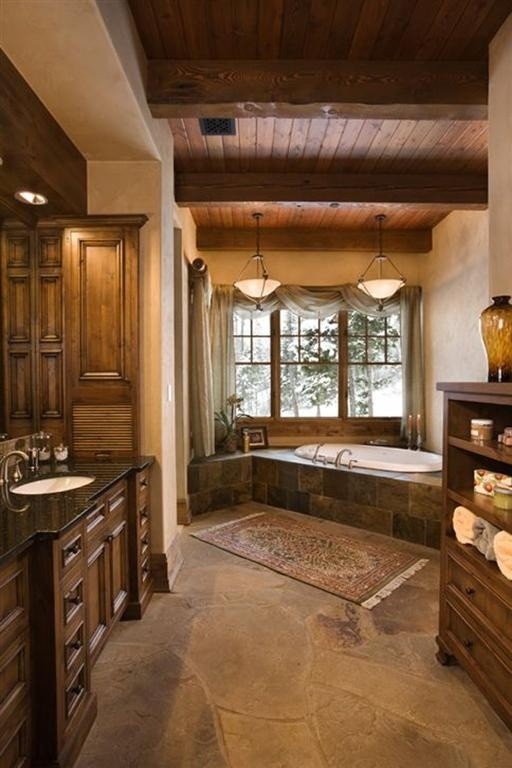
[187,509,431,612]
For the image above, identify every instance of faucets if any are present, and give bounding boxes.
[334,447,352,466]
[1,449,29,484]
[2,480,31,514]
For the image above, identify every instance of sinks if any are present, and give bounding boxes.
[9,471,95,495]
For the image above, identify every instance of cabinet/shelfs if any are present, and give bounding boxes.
[1,466,172,768]
[433,380,512,734]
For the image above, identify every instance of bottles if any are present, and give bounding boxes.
[470,418,496,441]
[31,431,52,461]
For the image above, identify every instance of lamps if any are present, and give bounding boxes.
[478,294,510,383]
[233,212,283,311]
[355,214,406,311]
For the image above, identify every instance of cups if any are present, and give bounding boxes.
[53,443,69,461]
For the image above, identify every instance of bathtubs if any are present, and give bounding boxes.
[295,443,443,473]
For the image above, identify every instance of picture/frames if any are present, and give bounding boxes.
[241,425,268,448]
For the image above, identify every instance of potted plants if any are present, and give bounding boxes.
[212,394,252,452]
